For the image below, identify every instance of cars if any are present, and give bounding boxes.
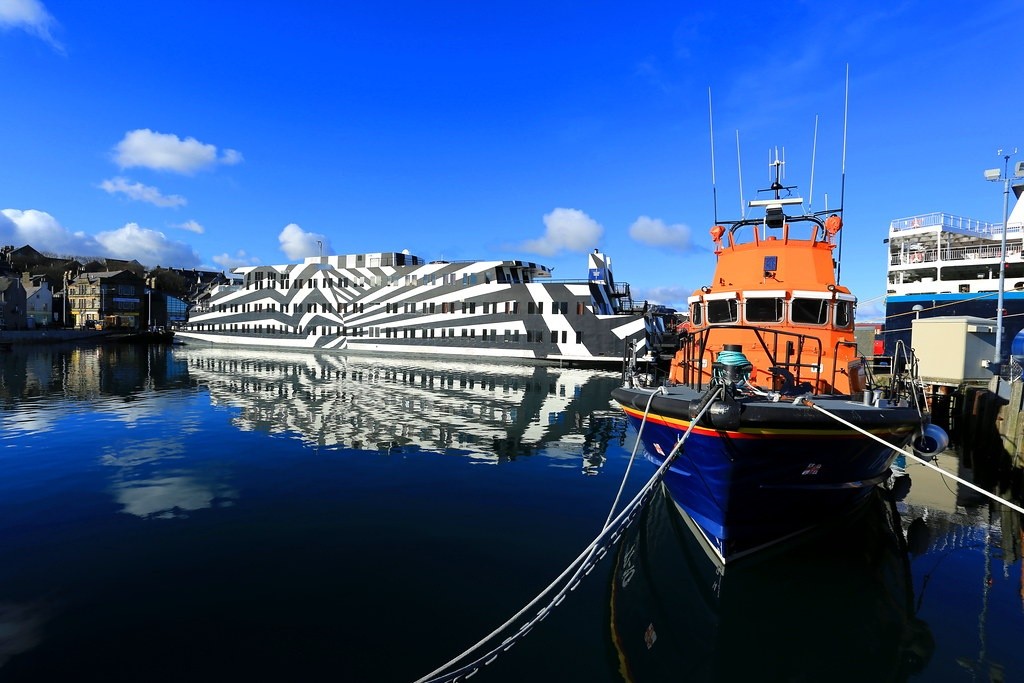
[85,319,99,329]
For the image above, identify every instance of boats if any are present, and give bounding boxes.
[173,252,656,364]
[611,146,919,585]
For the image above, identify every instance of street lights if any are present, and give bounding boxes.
[147,291,152,328]
[984,160,1024,362]
[102,288,115,313]
[63,271,68,329]
